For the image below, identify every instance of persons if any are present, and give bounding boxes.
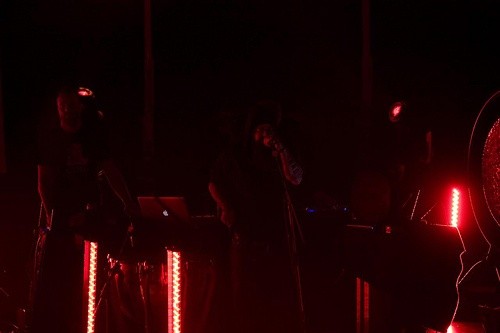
[28,90,138,311]
[207,112,303,285]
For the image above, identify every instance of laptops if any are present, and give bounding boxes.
[136,195,194,222]
